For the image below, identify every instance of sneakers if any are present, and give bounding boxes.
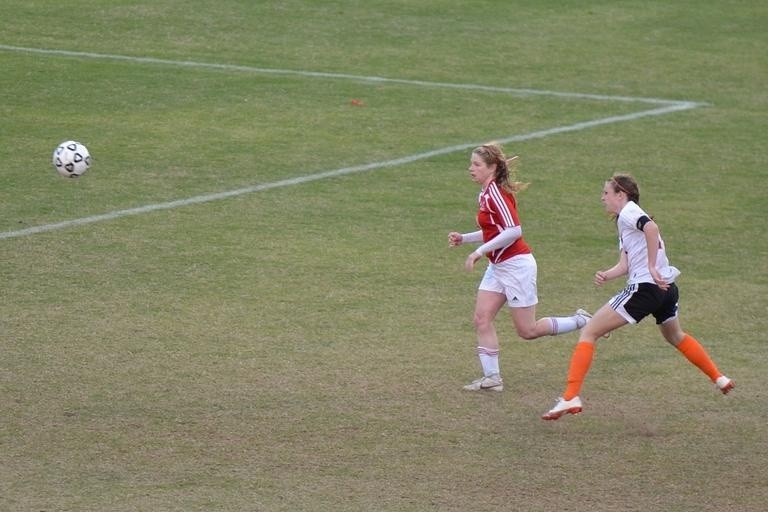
[541,395,583,421]
[461,377,505,393]
[714,373,735,394]
[575,308,610,338]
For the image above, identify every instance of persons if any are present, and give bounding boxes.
[447,140,610,391]
[542,172,734,420]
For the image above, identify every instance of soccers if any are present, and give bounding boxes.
[53,141,91,178]
